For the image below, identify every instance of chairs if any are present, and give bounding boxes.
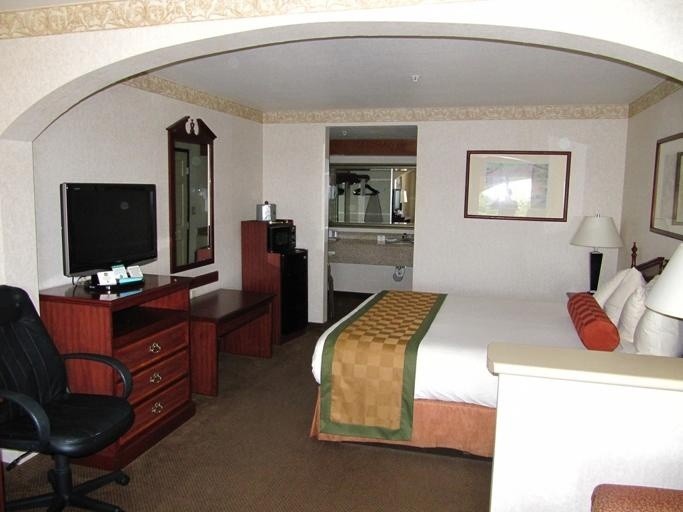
[0,285,136,512]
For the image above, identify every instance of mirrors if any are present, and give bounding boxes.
[166,115,217,273]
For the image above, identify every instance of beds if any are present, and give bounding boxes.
[312,256,668,457]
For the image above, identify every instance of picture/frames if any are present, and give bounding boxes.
[649,132,683,241]
[464,150,572,222]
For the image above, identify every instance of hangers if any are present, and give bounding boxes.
[352,178,379,196]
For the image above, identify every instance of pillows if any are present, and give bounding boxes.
[604,266,644,325]
[617,275,660,343]
[633,308,683,357]
[567,293,620,352]
[593,269,630,308]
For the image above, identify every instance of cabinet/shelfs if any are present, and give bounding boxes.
[241,220,308,345]
[38,273,196,472]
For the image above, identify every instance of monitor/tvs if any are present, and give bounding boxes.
[60,182,158,294]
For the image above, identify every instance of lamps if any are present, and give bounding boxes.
[570,210,625,295]
[644,242,683,321]
[190,288,277,397]
